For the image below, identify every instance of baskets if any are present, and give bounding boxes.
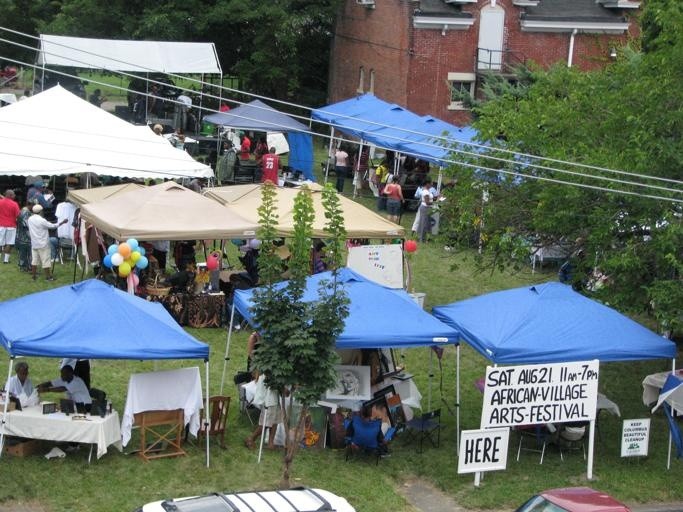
[144,271,171,296]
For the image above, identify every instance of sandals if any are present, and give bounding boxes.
[244,437,281,451]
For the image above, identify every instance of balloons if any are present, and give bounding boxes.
[403,240,417,253]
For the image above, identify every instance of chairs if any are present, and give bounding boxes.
[343,414,395,466]
[402,407,441,454]
[197,396,230,449]
[516,425,587,464]
[234,372,258,425]
[89,387,107,400]
[56,238,79,264]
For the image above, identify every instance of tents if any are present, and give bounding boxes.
[310,93,533,193]
[65,179,405,285]
[1,83,213,191]
[202,99,314,182]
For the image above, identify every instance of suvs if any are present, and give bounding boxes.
[133,484,359,512]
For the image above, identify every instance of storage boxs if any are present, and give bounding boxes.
[3,440,42,457]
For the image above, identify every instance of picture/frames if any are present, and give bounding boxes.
[285,348,414,448]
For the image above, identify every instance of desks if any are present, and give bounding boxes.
[183,136,232,156]
[512,235,572,276]
[595,392,620,441]
[0,406,122,466]
[241,374,266,424]
[642,368,683,416]
[147,261,264,328]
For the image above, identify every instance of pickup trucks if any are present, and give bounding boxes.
[509,485,636,512]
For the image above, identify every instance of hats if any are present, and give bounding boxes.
[32,204,42,213]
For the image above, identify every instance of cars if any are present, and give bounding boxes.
[123,71,181,117]
[28,62,91,103]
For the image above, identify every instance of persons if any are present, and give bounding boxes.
[34,365,92,453]
[245,367,289,452]
[559,238,594,287]
[88,89,282,188]
[4,361,36,401]
[336,372,359,394]
[1,172,81,281]
[61,356,90,389]
[245,328,264,410]
[341,405,388,458]
[556,422,586,447]
[144,239,371,332]
[521,422,549,449]
[321,126,444,245]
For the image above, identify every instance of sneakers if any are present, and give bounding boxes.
[30,275,56,281]
